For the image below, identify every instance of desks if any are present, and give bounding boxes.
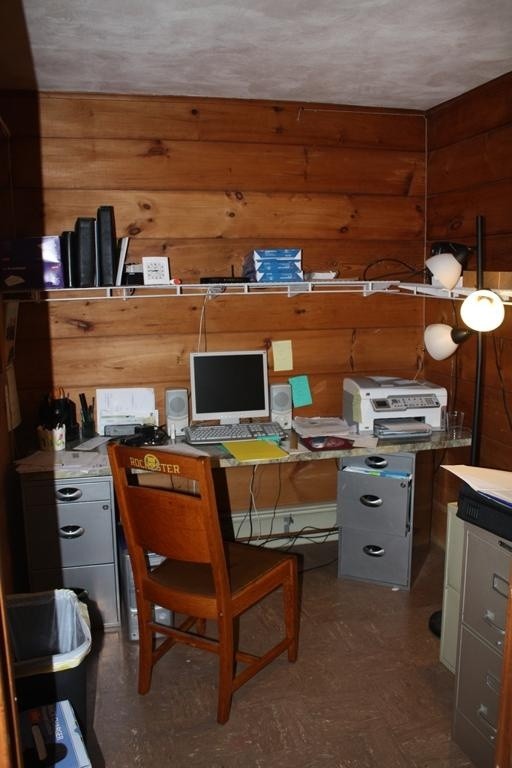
[125,415,477,549]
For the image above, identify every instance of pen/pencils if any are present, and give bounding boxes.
[47,387,69,429]
[79,392,94,424]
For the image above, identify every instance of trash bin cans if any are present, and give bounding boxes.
[5,588,92,744]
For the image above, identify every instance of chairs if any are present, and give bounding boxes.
[105,443,301,725]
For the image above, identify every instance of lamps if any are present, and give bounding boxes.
[422,212,505,639]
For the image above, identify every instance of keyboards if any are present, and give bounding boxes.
[186,423,286,445]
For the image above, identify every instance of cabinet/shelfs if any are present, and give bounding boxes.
[334,450,435,591]
[8,433,124,635]
[451,481,512,768]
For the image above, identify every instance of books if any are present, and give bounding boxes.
[60,229,77,287]
[291,414,351,439]
[114,236,130,287]
[96,205,116,287]
[73,216,99,288]
[14,447,107,475]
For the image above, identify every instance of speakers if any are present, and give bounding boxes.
[270,384,293,430]
[164,389,189,438]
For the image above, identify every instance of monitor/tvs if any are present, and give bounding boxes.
[190,351,268,424]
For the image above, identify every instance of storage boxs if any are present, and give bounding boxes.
[20,699,92,768]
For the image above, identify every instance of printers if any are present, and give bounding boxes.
[343,376,448,442]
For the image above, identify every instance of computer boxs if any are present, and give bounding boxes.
[118,529,173,642]
